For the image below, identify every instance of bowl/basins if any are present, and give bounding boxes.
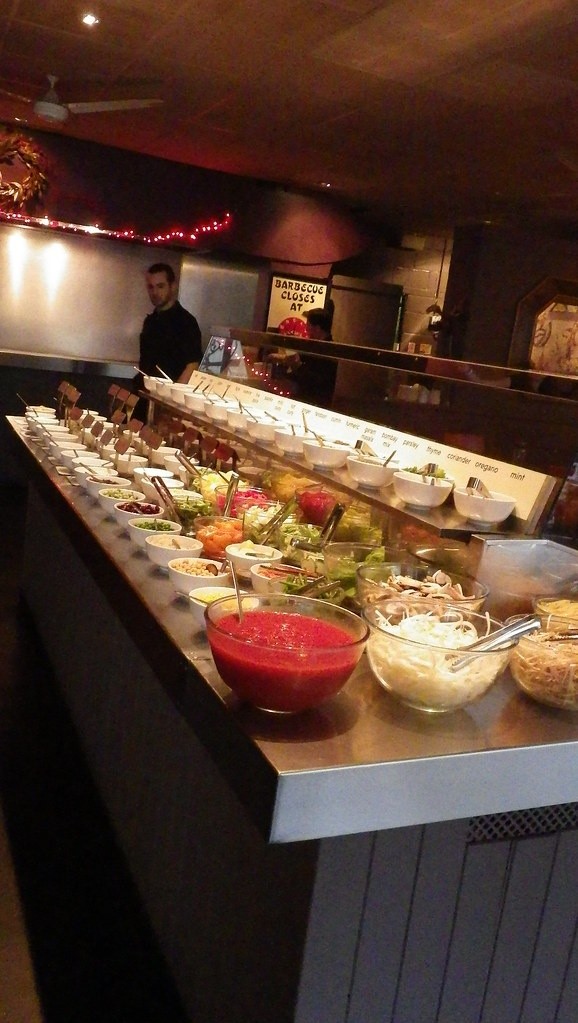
[505,613,577,715]
[361,597,521,715]
[23,377,578,637]
[207,598,369,714]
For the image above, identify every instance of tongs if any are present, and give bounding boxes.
[446,616,543,674]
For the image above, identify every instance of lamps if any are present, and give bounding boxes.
[425,237,448,317]
[83,13,99,27]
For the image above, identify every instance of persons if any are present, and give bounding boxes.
[266,307,339,410]
[126,262,204,427]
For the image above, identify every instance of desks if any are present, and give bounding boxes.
[7,416,578,844]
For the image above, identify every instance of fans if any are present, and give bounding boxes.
[0,75,165,125]
